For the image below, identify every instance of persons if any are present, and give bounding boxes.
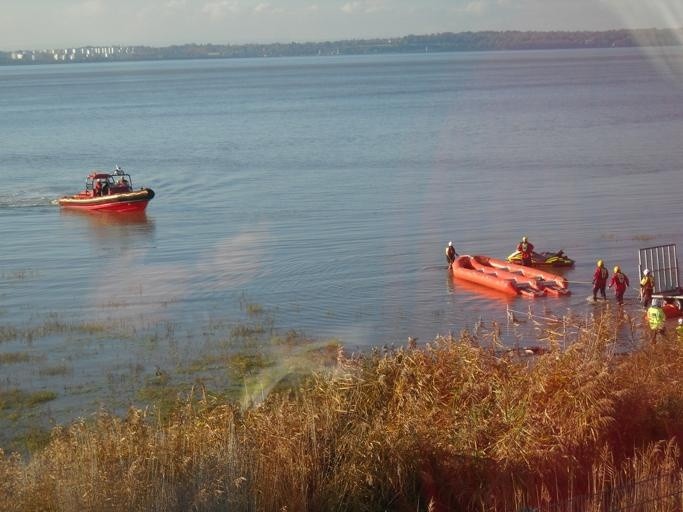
[593,260,609,303]
[516,237,534,267]
[96,178,103,197]
[116,176,128,185]
[639,268,655,309]
[446,241,457,271]
[608,265,630,305]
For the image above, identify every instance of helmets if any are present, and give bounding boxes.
[614,265,622,273]
[678,318,683,326]
[596,258,603,266]
[642,268,651,277]
[523,236,529,243]
[651,298,661,307]
[448,241,455,249]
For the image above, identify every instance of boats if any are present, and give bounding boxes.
[451,250,576,300]
[57,164,155,214]
